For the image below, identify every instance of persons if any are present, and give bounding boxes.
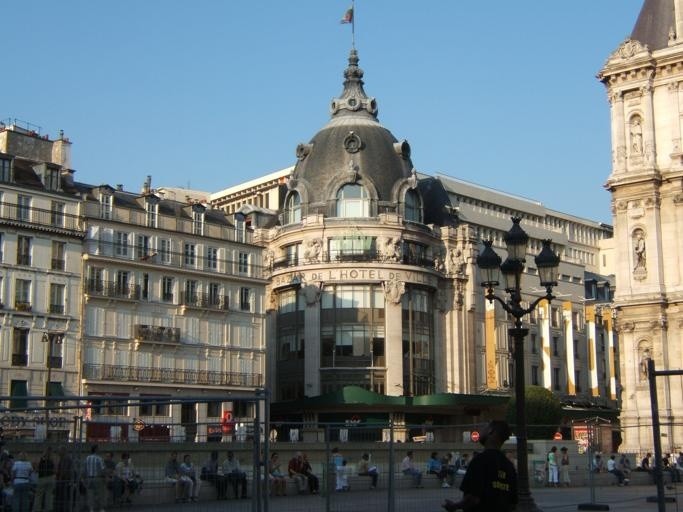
[632,233,647,267]
[630,120,644,151]
[1,418,469,512]
[547,446,683,489]
[441,420,518,512]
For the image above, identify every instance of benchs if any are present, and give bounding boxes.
[130,470,683,506]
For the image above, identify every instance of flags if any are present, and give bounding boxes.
[340,6,353,24]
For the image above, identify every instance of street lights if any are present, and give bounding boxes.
[41,330,64,440]
[474,215,561,512]
